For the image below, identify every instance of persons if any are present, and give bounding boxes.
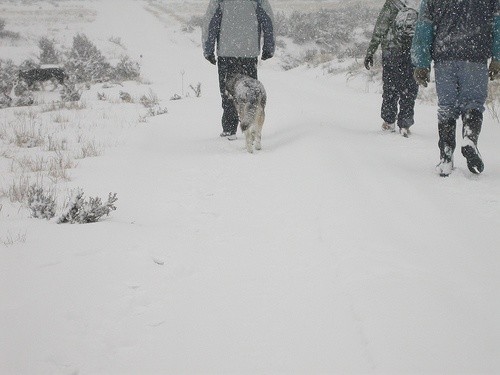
[202,0,275,142]
[409,0,500,177]
[364,0,423,137]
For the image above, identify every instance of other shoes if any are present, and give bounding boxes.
[220,131,237,140]
[400,127,411,138]
[382,121,396,132]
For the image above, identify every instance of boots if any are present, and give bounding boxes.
[438,118,456,177]
[460,109,485,175]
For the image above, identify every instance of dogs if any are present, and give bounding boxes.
[224,71,267,154]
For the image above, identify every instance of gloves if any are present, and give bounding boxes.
[364,54,374,70]
[413,67,430,87]
[489,58,500,80]
[261,50,272,60]
[206,54,217,65]
